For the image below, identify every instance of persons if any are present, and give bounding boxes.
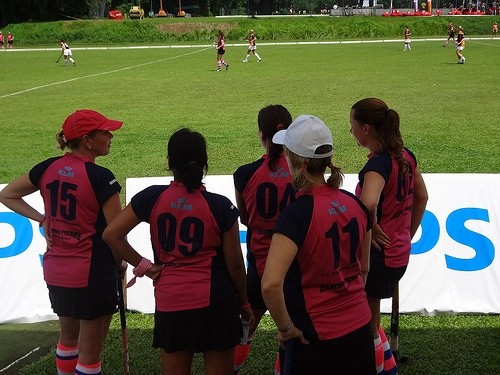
[402,25,412,52]
[0,31,5,50]
[7,32,14,50]
[492,22,498,40]
[60,38,76,67]
[0,109,132,375]
[421,2,426,14]
[102,128,254,375]
[215,30,230,71]
[441,22,457,47]
[454,26,466,65]
[242,29,262,62]
[232,104,293,375]
[349,98,429,375]
[260,115,377,375]
[448,0,500,15]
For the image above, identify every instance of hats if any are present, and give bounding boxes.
[272,115,334,157]
[62,110,122,141]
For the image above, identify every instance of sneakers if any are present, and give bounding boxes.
[243,60,249,63]
[226,65,229,70]
[216,70,221,71]
[257,58,261,62]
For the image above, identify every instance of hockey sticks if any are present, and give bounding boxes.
[117,271,130,375]
[55,53,63,64]
[391,280,409,362]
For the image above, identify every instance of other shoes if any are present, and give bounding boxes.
[73,62,76,67]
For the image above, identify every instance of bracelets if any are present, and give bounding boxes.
[38,216,46,227]
[239,302,250,309]
[124,257,151,289]
[278,320,291,333]
